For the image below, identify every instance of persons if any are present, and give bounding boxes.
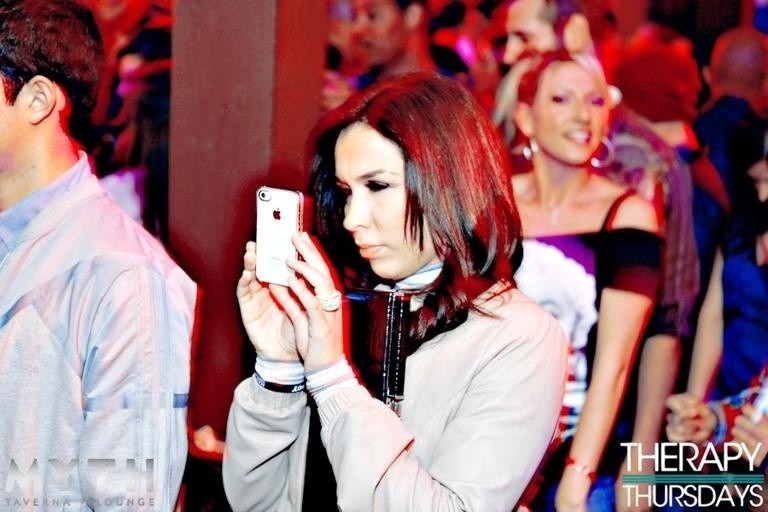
[2,0,767,510]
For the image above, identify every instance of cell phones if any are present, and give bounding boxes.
[254,185,304,288]
